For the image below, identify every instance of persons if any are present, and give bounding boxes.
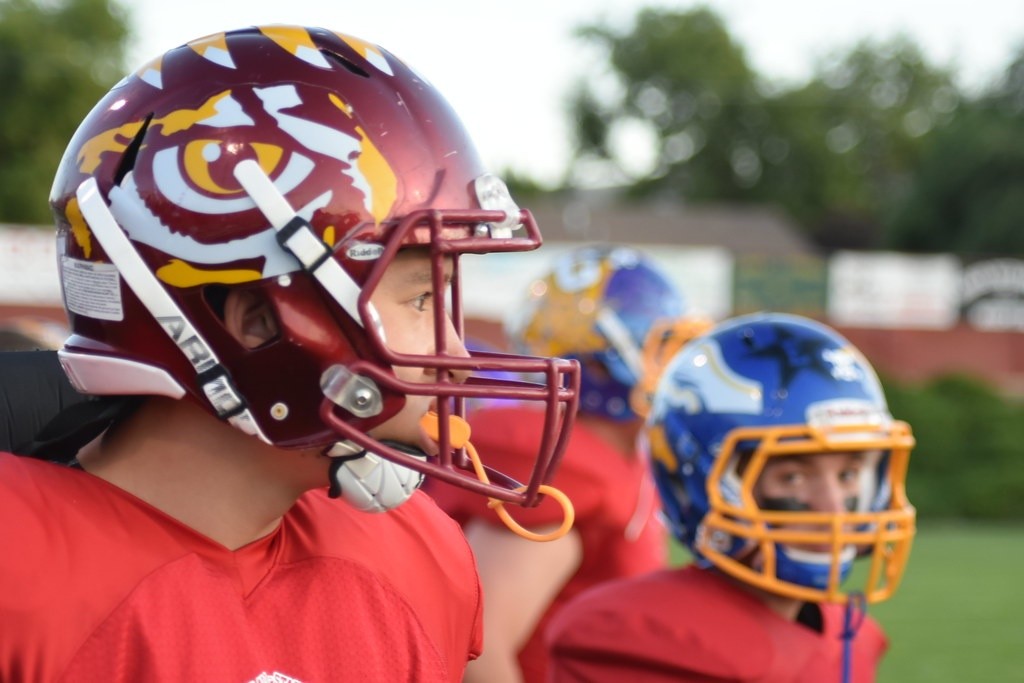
[549,310,918,683]
[1,20,540,683]
[414,243,715,680]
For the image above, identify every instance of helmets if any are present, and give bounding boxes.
[52,27,492,450]
[634,311,893,588]
[521,243,708,419]
